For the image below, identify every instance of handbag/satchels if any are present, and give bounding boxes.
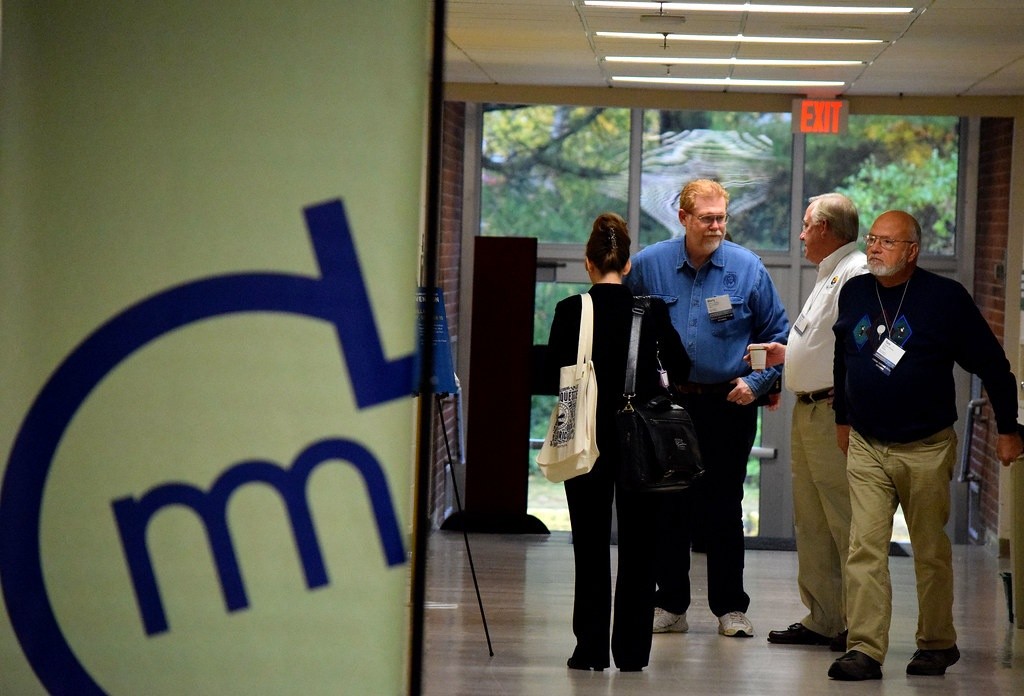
[617,297,707,491]
[536,291,600,483]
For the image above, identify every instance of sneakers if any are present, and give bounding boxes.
[768,622,833,646]
[653,607,689,633]
[717,610,753,637]
[829,629,849,651]
[906,643,960,676]
[828,648,883,680]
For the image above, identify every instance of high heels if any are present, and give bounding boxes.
[567,653,605,671]
[618,666,644,672]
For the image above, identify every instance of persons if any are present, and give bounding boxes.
[745,193,870,652]
[827,211,1024,681]
[623,179,789,637]
[548,213,693,671]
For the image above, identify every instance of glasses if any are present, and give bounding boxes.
[686,211,731,225]
[862,232,914,251]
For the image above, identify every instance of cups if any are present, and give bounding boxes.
[749,344,767,370]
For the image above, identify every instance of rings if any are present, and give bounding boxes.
[739,399,742,404]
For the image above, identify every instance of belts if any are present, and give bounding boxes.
[797,386,836,404]
